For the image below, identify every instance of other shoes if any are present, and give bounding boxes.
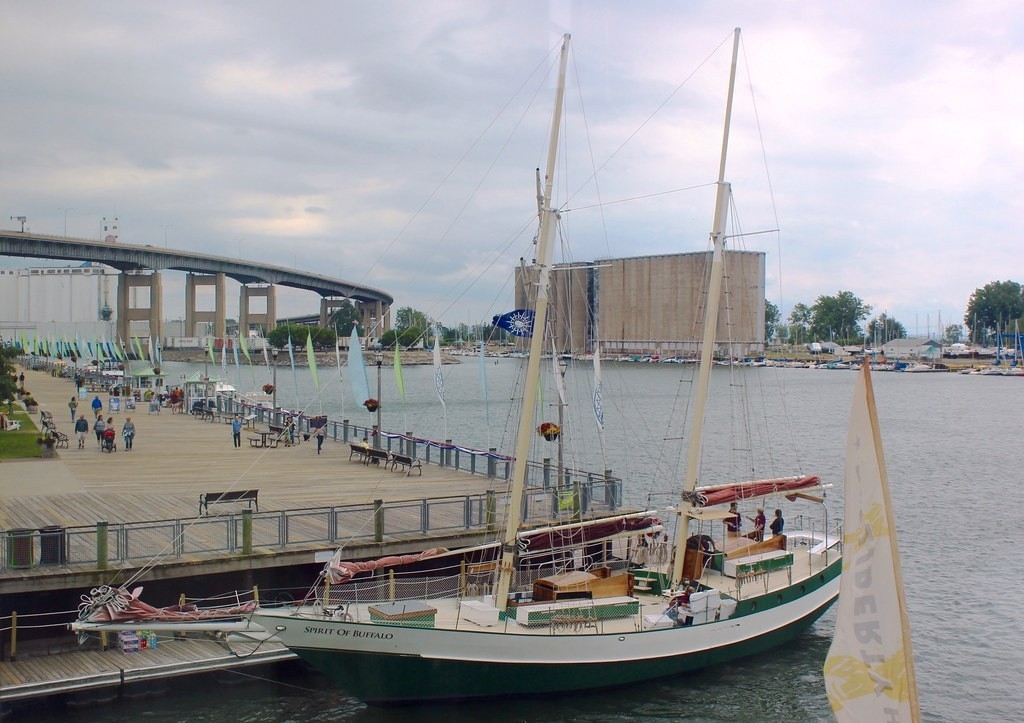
[97,446,99,448]
[372,461,381,464]
[82,445,84,447]
[319,447,322,450]
[292,444,295,446]
[285,443,290,447]
[172,412,177,414]
[235,447,237,450]
[101,444,103,446]
[125,448,129,451]
[238,447,240,449]
[130,449,132,451]
[179,411,183,413]
[72,420,75,422]
[79,444,81,448]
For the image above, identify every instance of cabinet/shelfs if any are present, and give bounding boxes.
[459,601,500,627]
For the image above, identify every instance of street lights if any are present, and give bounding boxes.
[8,336,128,386]
[177,315,184,351]
[554,359,567,489]
[268,346,282,426]
[156,341,164,398]
[202,343,210,396]
[374,348,385,450]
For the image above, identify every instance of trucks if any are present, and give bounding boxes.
[164,331,276,354]
[337,337,382,350]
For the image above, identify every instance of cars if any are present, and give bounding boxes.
[283,344,294,354]
[295,345,301,352]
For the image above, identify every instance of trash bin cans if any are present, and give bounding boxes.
[39,524,67,564]
[6,528,35,567]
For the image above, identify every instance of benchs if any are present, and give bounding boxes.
[348,444,422,476]
[194,407,248,425]
[290,431,301,445]
[39,410,70,448]
[199,489,259,516]
[247,437,260,447]
[267,438,281,448]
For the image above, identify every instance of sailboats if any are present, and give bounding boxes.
[61,30,841,706]
[437,335,1024,379]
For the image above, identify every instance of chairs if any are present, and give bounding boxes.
[3,415,21,432]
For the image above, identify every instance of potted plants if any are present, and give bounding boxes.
[16,387,38,413]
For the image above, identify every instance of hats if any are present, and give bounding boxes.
[757,507,764,512]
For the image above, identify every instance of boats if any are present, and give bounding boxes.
[185,372,237,399]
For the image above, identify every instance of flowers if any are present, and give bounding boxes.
[362,397,379,407]
[262,384,275,391]
[535,421,559,436]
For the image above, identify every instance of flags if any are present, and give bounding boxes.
[493,309,549,339]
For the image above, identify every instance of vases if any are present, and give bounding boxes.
[367,406,378,412]
[265,390,272,393]
[544,434,556,440]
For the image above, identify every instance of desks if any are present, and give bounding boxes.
[269,425,288,435]
[255,432,274,447]
[201,409,218,414]
[228,411,244,417]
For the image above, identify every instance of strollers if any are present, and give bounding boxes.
[98,429,117,453]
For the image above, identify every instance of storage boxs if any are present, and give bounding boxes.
[644,615,673,630]
[719,599,737,616]
[118,630,155,653]
[677,588,721,625]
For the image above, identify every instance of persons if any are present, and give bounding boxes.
[770,509,784,538]
[19,373,25,388]
[74,415,90,449]
[313,426,327,455]
[360,438,379,464]
[282,416,297,448]
[668,586,695,628]
[94,415,106,449]
[232,415,242,449]
[74,377,84,392]
[103,418,116,433]
[746,507,766,542]
[160,386,185,414]
[723,502,743,538]
[92,396,103,420]
[122,418,136,452]
[68,396,78,423]
